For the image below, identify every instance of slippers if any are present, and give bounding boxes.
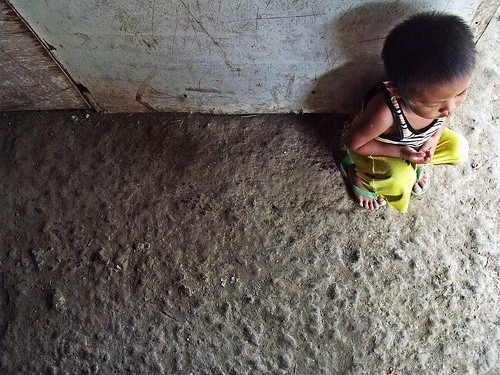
[412,165,432,195]
[339,156,385,208]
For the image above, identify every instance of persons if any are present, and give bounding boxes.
[339,10,478,212]
